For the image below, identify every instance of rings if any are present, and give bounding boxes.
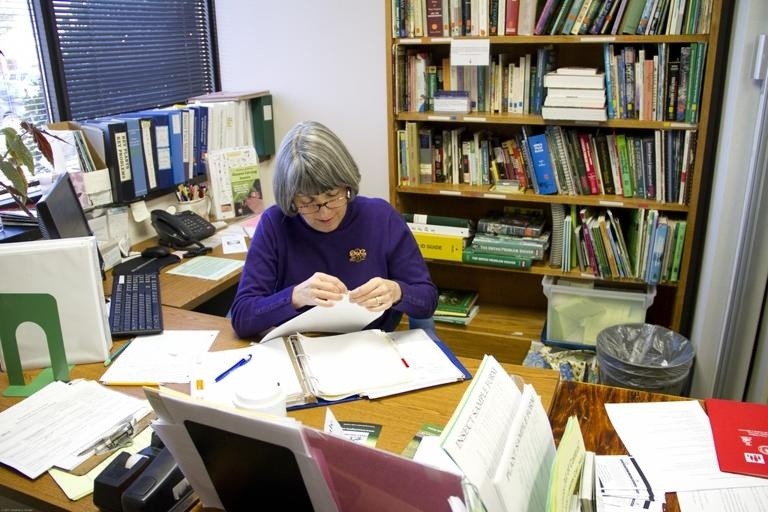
[376,296,382,306]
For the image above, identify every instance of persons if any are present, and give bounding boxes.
[232,121,439,343]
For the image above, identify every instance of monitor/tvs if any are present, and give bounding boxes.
[35,171,105,281]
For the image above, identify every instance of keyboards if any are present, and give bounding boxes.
[107,274,164,336]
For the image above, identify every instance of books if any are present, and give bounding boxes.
[191,330,474,423]
[397,125,698,326]
[400,353,768,511]
[2,86,279,236]
[392,0,713,123]
[0,235,113,372]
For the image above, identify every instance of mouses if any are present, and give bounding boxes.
[140,246,168,261]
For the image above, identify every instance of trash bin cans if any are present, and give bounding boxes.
[595,323,696,396]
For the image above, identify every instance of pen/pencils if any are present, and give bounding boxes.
[102,336,135,366]
[176,184,206,202]
[214,353,253,381]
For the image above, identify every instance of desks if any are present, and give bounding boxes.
[99,216,256,311]
[548,379,767,509]
[2,288,561,510]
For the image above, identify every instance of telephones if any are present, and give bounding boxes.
[151,209,215,247]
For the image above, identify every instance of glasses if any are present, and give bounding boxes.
[290,188,351,214]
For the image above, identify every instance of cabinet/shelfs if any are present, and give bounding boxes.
[382,0,724,362]
